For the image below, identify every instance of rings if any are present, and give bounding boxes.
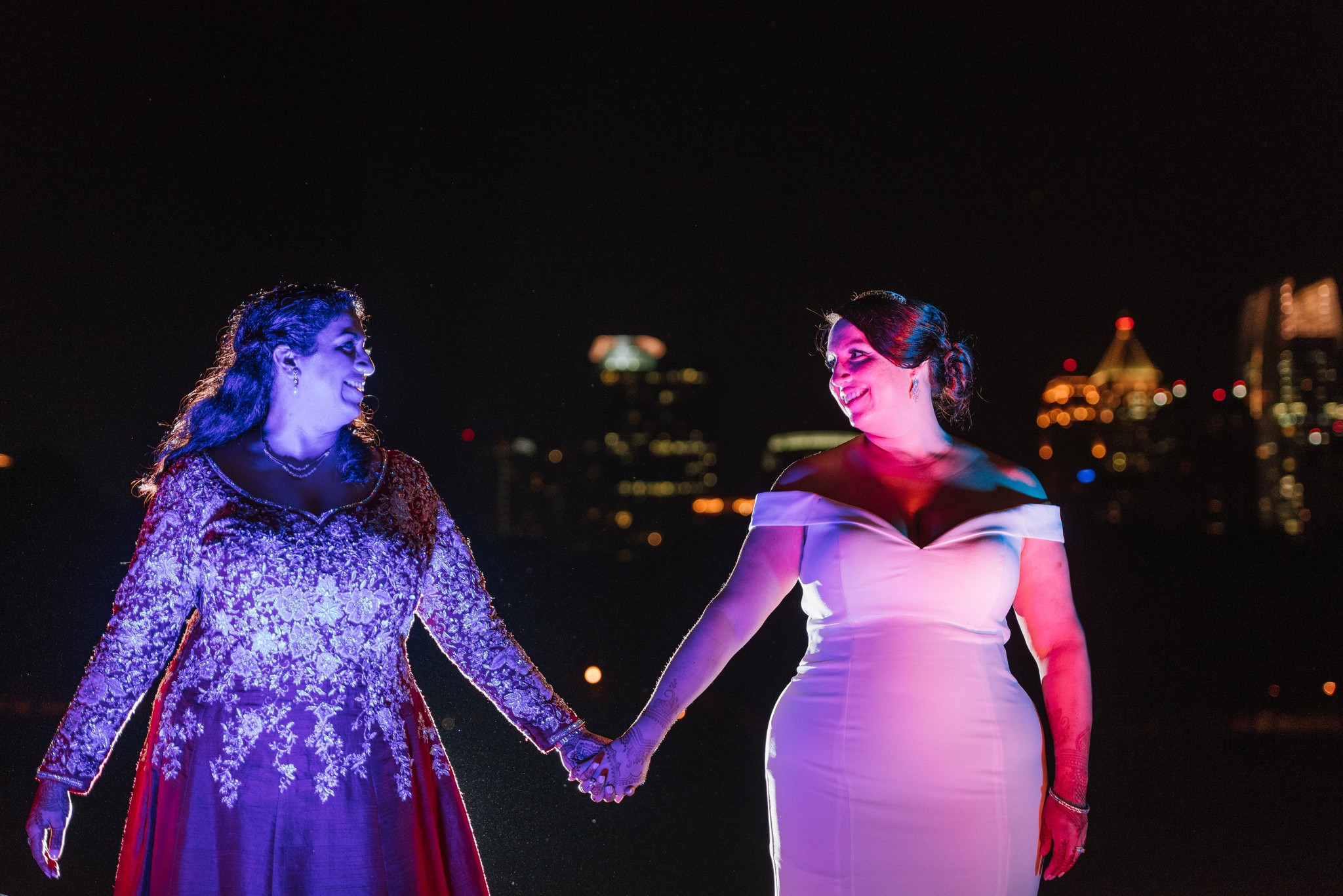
[27,837,31,846]
[1076,845,1086,853]
[586,779,595,789]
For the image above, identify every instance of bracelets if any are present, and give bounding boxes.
[1049,787,1090,814]
[554,727,588,753]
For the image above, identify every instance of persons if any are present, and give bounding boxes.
[568,288,1093,896]
[25,284,622,895]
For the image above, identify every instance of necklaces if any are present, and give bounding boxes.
[260,424,337,478]
